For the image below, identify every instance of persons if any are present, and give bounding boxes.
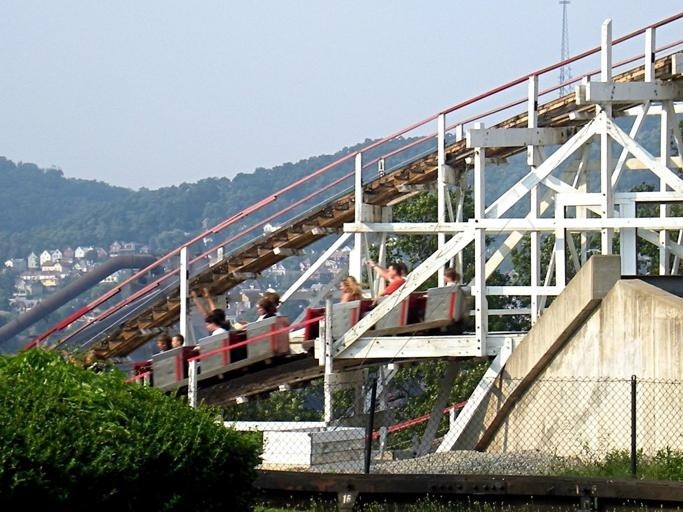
[340,259,460,302]
[158,288,279,351]
